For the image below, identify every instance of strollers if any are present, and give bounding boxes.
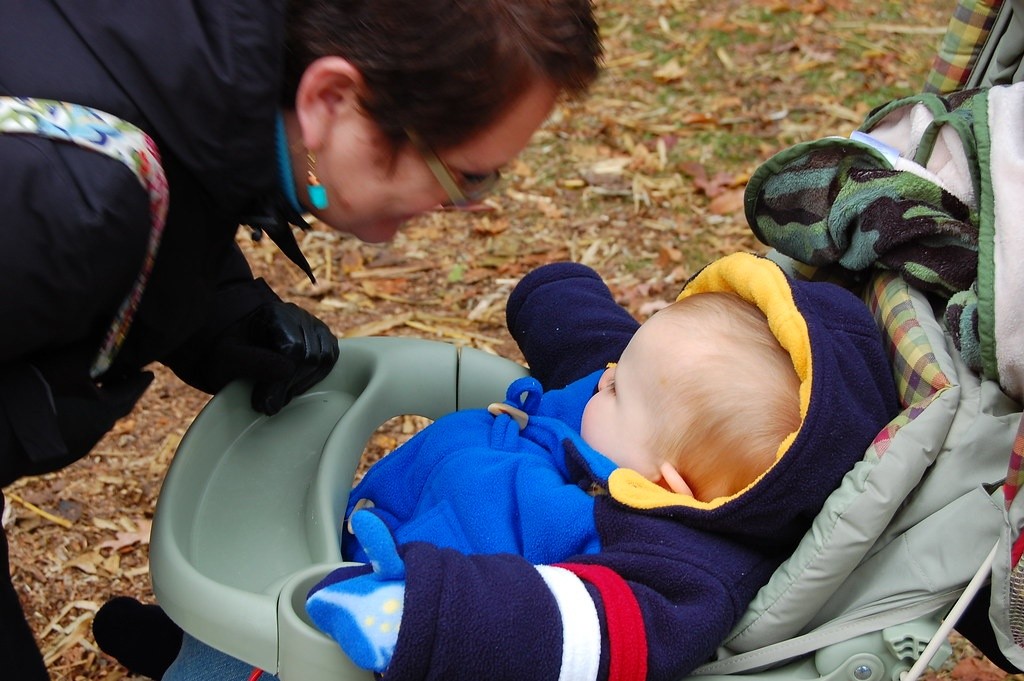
[149,88,1024,681]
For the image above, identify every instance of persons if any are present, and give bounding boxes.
[91,250,902,681]
[0,0,612,681]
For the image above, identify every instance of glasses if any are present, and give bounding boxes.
[397,121,499,209]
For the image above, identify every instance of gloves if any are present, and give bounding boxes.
[306,509,406,669]
